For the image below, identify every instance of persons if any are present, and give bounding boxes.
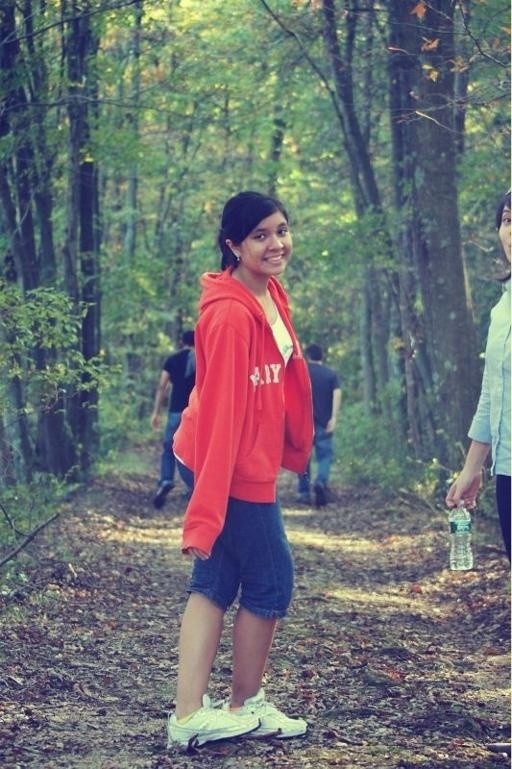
[167,191,314,750]
[445,187,511,561]
[296,342,342,506]
[150,330,196,509]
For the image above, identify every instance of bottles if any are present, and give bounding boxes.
[448,499,474,571]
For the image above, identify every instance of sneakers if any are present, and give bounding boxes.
[152,479,176,510]
[166,687,310,749]
[295,480,327,508]
